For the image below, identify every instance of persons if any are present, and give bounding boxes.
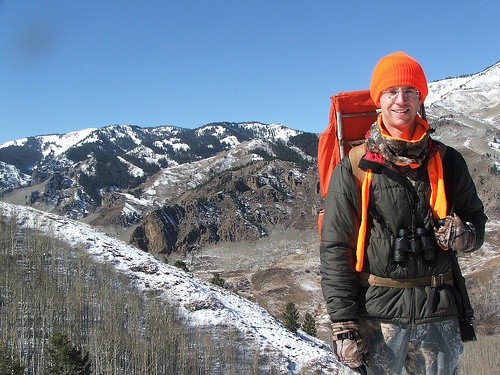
[319,52,488,375]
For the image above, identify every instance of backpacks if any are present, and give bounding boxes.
[318,90,453,288]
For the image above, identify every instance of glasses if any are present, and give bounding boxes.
[381,86,420,98]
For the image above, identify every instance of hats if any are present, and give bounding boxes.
[369,52,428,108]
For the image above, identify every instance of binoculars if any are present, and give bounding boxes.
[394,229,434,262]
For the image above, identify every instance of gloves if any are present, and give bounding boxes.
[434,213,477,252]
[331,321,373,375]
